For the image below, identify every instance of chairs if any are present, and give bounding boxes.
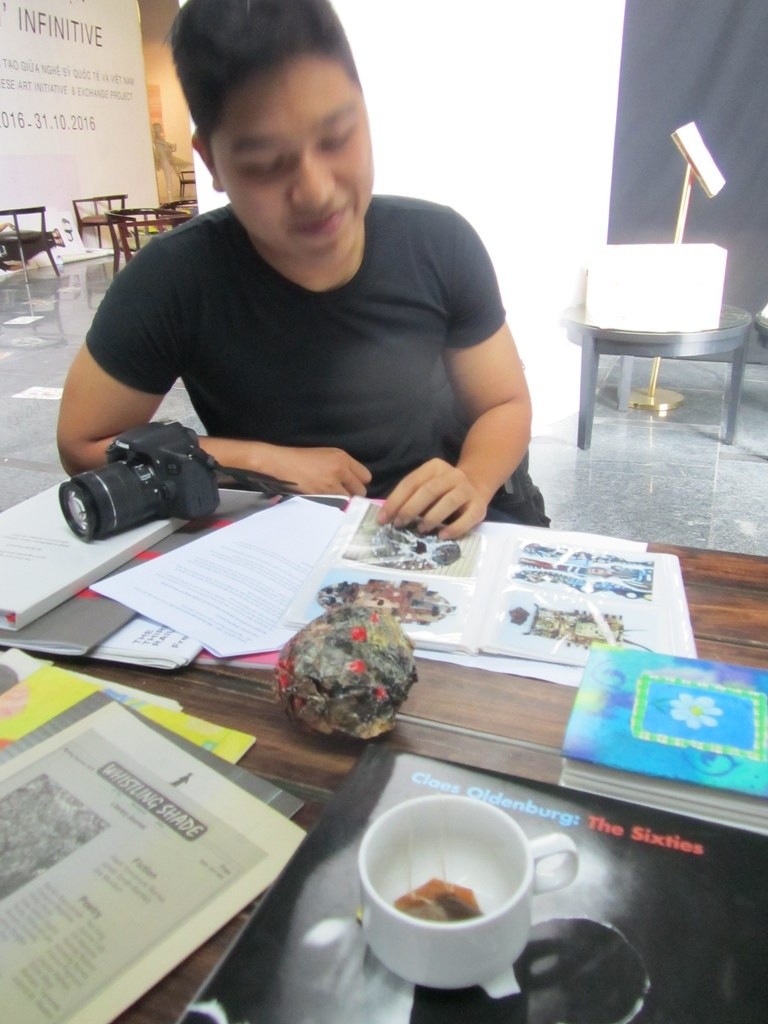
[73,194,198,279]
[173,164,196,201]
[0,207,60,284]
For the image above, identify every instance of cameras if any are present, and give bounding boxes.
[59,419,220,545]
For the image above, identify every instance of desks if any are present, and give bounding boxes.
[564,302,752,450]
[0,540,768,1024]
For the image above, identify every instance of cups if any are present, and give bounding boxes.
[357,793,580,990]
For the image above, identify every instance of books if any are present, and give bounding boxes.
[177,744,768,1024]
[279,495,655,667]
[0,665,307,1024]
[0,478,348,672]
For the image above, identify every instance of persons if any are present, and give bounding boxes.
[56,0,521,541]
